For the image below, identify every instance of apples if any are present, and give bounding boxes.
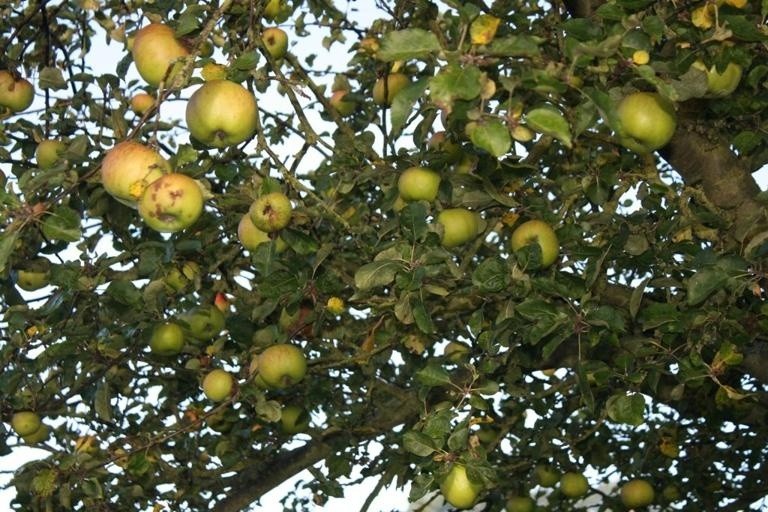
[262,0,287,20]
[1,25,744,512]
